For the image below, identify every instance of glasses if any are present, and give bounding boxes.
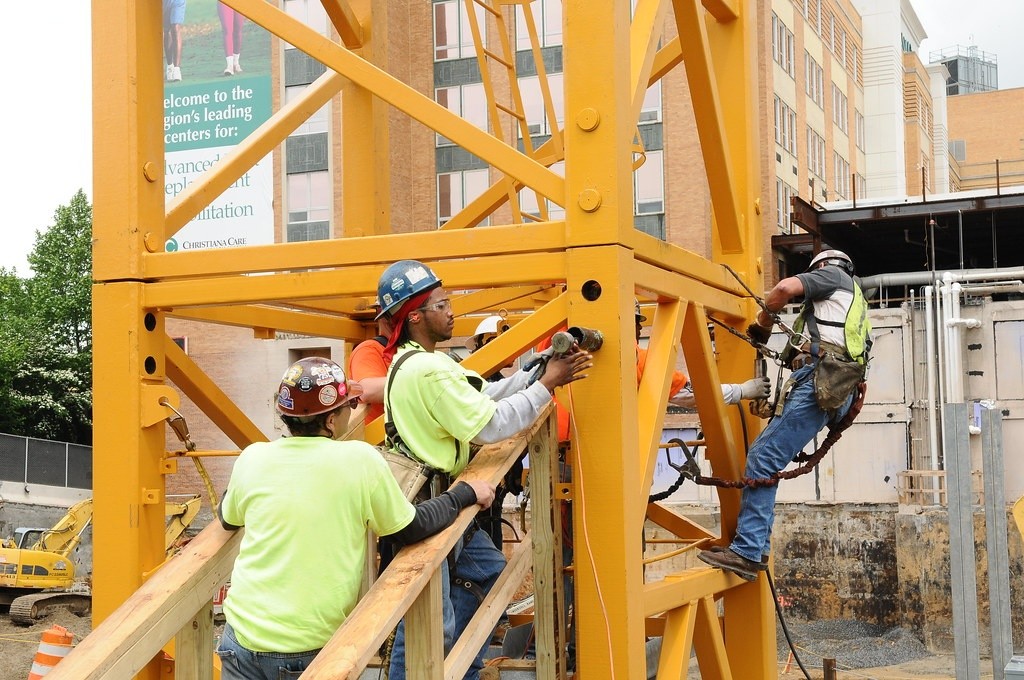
[414,299,454,312]
[337,396,359,412]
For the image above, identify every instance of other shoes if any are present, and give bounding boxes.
[697,546,769,581]
[478,666,500,680]
[490,622,513,645]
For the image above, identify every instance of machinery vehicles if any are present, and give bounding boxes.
[0,492,203,617]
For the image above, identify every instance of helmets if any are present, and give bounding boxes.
[374,260,442,321]
[368,294,381,309]
[803,250,852,273]
[635,297,646,322]
[276,356,363,417]
[464,316,504,349]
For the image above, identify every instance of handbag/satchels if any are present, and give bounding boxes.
[374,445,433,504]
[814,353,866,412]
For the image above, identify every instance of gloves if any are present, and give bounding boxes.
[745,311,772,345]
[739,377,771,400]
[749,398,775,419]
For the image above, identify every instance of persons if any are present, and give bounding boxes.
[345,258,772,680]
[216,354,496,680]
[698,248,871,585]
[161,0,243,81]
